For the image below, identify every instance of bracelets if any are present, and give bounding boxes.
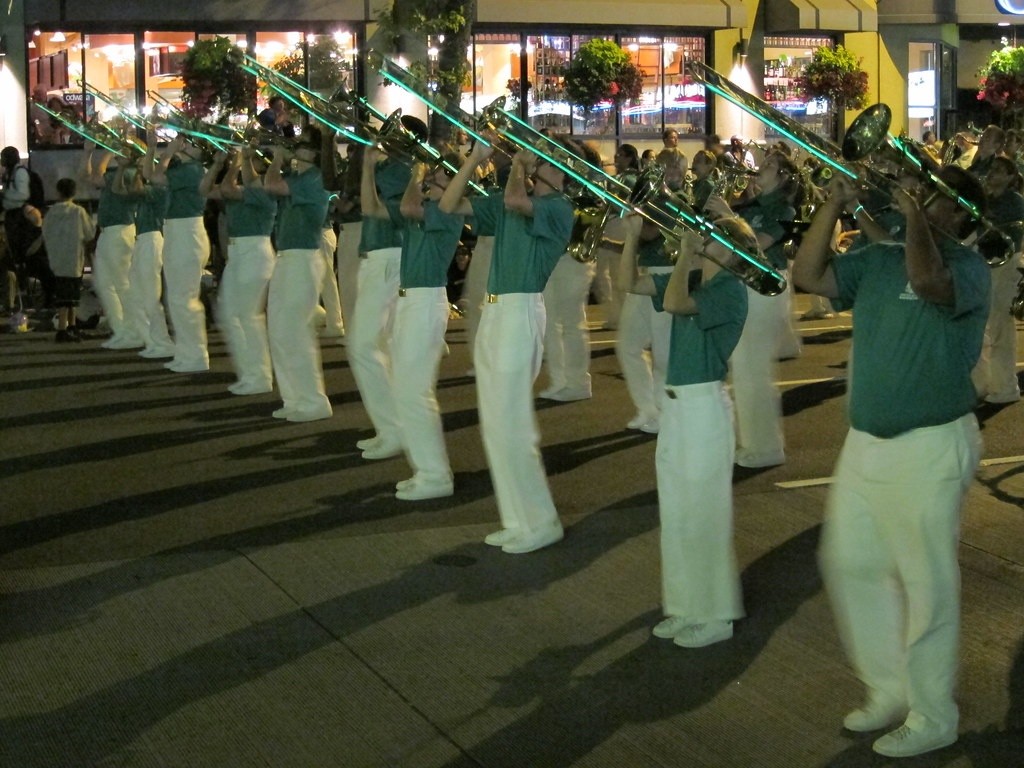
[852,204,864,219]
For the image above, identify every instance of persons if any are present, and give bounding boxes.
[244,80,1024,555]
[141,123,214,373]
[113,120,176,361]
[198,133,278,395]
[605,196,758,648]
[790,161,996,757]
[80,131,149,351]
[0,146,110,342]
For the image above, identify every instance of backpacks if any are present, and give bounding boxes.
[13,166,44,208]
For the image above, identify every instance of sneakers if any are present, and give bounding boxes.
[272,408,332,422]
[873,705,959,757]
[627,411,663,435]
[843,702,910,732]
[138,345,174,358]
[652,615,734,647]
[227,381,273,395]
[485,519,563,553]
[395,473,454,501]
[163,357,210,372]
[537,309,831,401]
[735,448,786,468]
[102,335,145,349]
[357,436,402,459]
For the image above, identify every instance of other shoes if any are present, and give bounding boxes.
[56,331,81,342]
[467,368,475,376]
[67,326,89,340]
[985,391,1020,404]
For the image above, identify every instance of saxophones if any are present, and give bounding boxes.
[661,164,758,259]
[742,139,766,166]
[569,167,644,264]
[942,134,977,170]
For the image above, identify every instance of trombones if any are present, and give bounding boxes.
[484,105,786,299]
[687,57,1015,273]
[361,46,610,218]
[226,43,415,164]
[31,78,293,177]
